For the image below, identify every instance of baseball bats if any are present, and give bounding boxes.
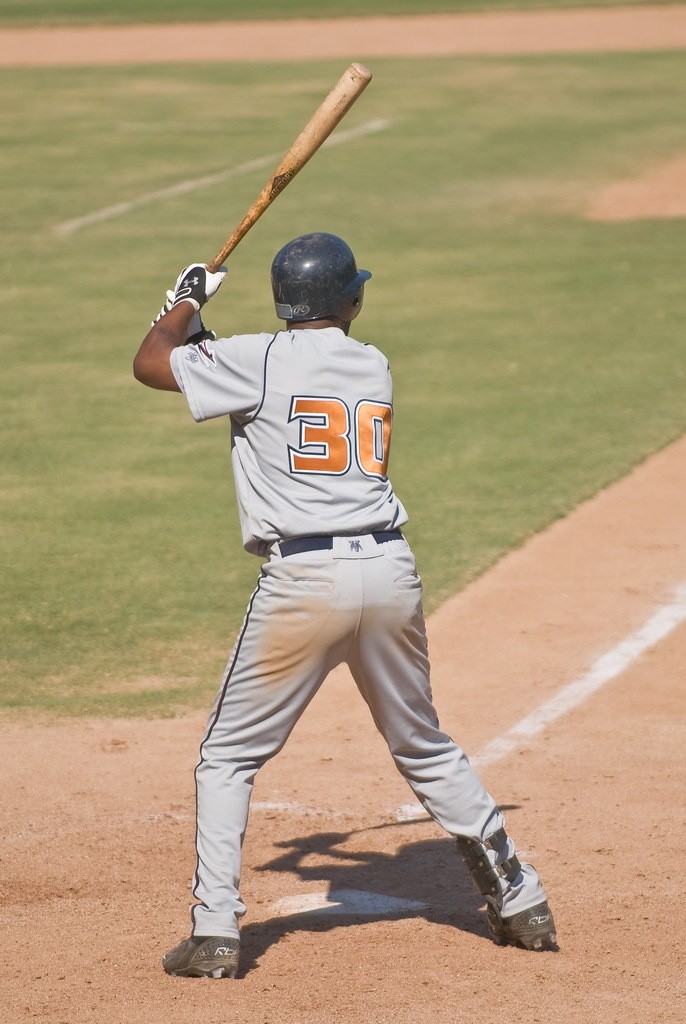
[201,59,377,276]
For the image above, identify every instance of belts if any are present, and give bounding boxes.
[278,529,404,559]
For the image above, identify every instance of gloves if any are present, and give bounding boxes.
[151,290,217,346]
[171,262,228,314]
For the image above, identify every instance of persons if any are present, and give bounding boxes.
[130,233,557,978]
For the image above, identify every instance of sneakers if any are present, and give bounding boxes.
[488,900,557,949]
[161,936,239,979]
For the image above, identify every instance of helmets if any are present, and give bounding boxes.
[270,233,372,322]
